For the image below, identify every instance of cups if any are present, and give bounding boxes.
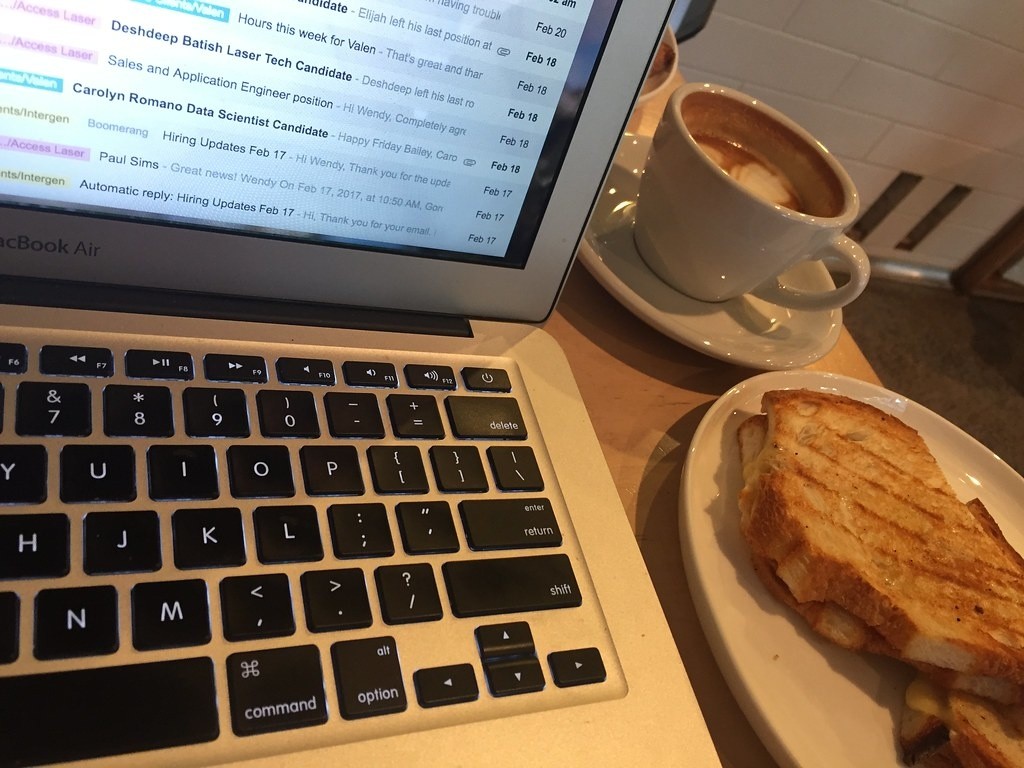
[631,81,871,314]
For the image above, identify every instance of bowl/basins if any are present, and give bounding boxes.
[634,22,680,101]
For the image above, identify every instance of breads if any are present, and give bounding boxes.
[737,388,1024,768]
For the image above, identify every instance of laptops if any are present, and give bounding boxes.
[0,0,725,768]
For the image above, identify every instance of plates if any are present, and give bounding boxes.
[577,134,842,369]
[678,369,1024,768]
